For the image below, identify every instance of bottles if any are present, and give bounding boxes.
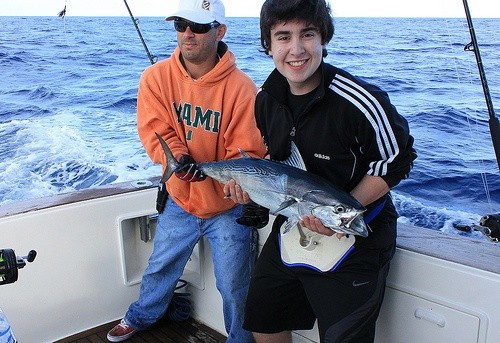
[0,306,18,343]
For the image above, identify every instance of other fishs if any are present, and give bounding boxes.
[152,131,369,239]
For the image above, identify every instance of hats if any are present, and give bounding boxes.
[165,0,225,25]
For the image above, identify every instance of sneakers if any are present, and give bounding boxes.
[107,318,137,343]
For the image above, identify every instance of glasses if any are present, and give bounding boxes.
[174,19,219,34]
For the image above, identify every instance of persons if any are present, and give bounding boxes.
[107,0,268,343]
[224,0,417,343]
